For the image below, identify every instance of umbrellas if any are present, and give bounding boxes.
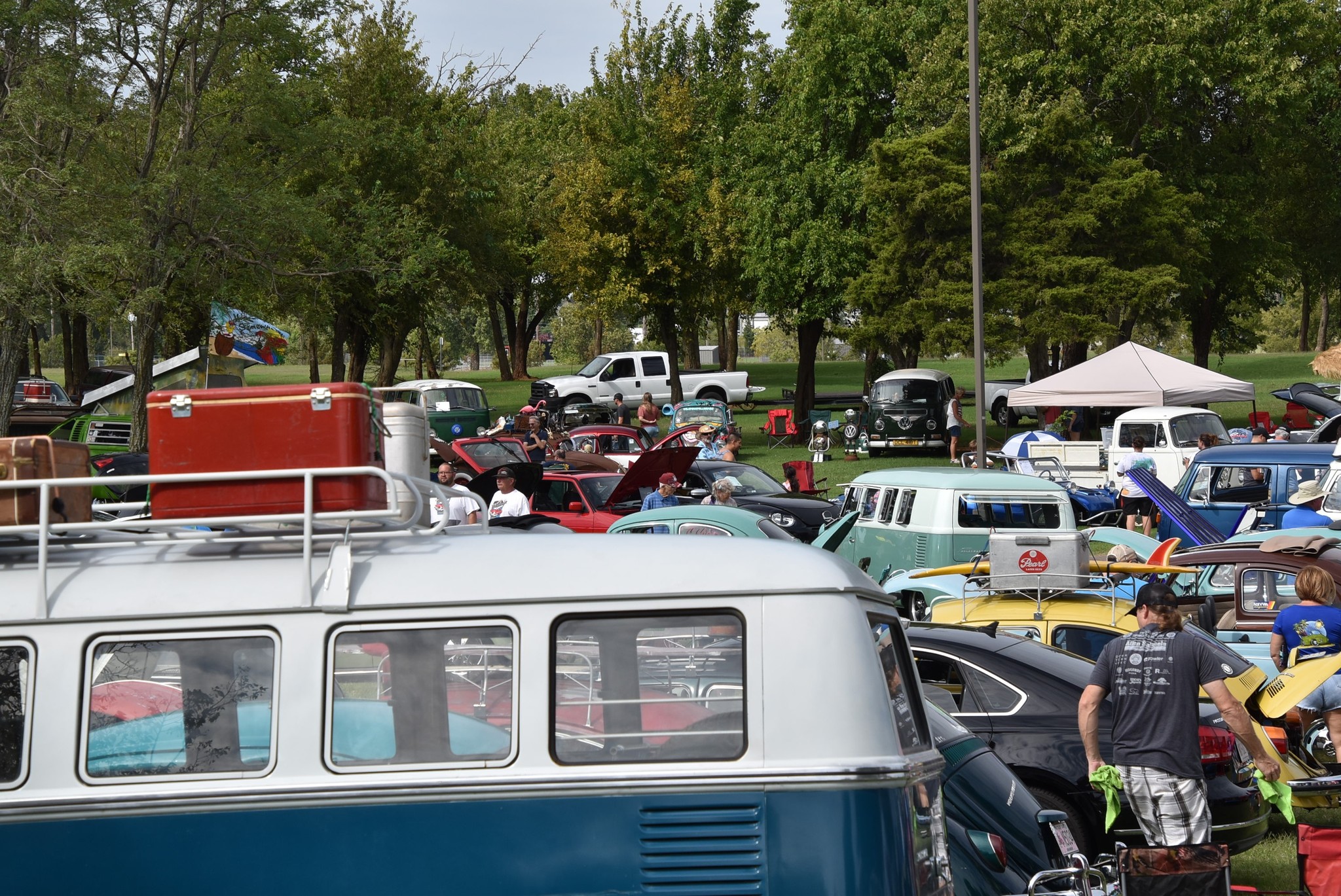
[996,429,1066,461]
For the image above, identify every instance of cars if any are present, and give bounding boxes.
[6,366,151,524]
[1079,381,1341,606]
[908,527,1341,895]
[363,378,837,548]
[862,367,964,458]
[605,504,861,553]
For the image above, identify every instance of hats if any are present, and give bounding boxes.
[1276,424,1291,440]
[491,467,516,478]
[1124,582,1179,617]
[695,425,716,441]
[659,472,682,488]
[1106,544,1138,563]
[1252,426,1273,439]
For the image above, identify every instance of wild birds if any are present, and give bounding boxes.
[519,400,547,415]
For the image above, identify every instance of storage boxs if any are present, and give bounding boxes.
[514,414,543,432]
[988,527,1096,589]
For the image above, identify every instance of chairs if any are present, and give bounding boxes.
[1246,412,1286,434]
[533,493,558,510]
[797,409,831,448]
[1231,822,1341,896]
[782,460,831,501]
[1282,402,1317,431]
[759,409,799,450]
[837,411,868,448]
[1216,599,1260,630]
[1116,842,1232,896]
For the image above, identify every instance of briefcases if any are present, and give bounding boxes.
[23,378,51,404]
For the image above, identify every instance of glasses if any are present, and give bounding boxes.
[614,398,617,400]
[438,472,454,474]
[553,456,558,458]
[528,423,539,426]
[701,432,712,436]
[1131,560,1138,564]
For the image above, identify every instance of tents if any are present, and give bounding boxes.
[1004,340,1258,444]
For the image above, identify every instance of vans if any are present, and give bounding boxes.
[817,466,1094,621]
[0,467,955,896]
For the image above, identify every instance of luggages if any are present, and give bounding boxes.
[515,407,550,431]
[0,380,430,527]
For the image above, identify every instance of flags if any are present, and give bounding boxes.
[210,296,291,367]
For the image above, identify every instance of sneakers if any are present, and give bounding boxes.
[950,458,960,463]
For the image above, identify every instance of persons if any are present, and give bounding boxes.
[1041,405,1062,433]
[968,439,994,469]
[612,393,631,452]
[487,467,530,520]
[1243,426,1272,488]
[1265,425,1299,504]
[542,449,577,472]
[1117,436,1157,536]
[640,472,682,534]
[1269,565,1341,764]
[1065,405,1085,441]
[1281,479,1335,529]
[1182,434,1220,470]
[715,432,742,462]
[946,386,972,464]
[700,478,738,507]
[428,462,481,529]
[523,416,548,464]
[1077,582,1281,846]
[637,392,660,446]
[782,465,800,492]
[1073,543,1150,598]
[692,424,719,460]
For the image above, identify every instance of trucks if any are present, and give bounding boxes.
[1014,406,1234,505]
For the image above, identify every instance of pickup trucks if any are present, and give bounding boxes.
[984,360,1125,429]
[528,350,766,415]
